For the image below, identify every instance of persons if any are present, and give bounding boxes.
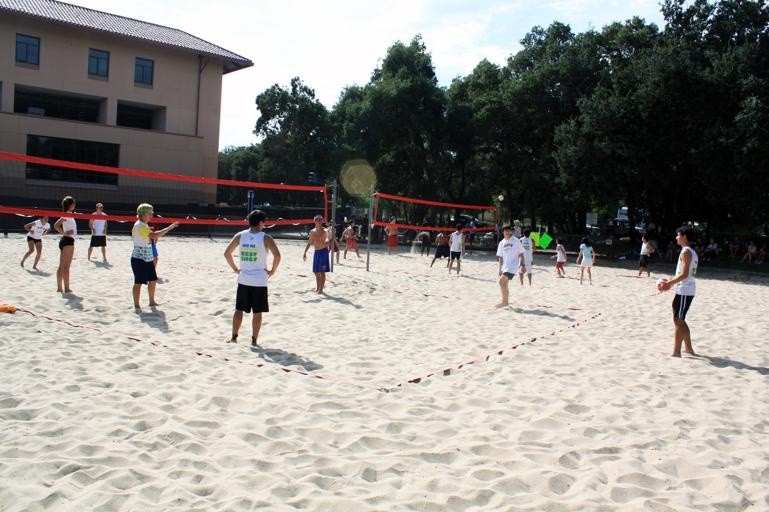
[576,237,595,285]
[303,215,331,294]
[186,213,197,220]
[130,203,180,308]
[637,233,653,277]
[405,219,536,308]
[54,196,77,292]
[149,203,179,268]
[550,237,567,277]
[326,219,340,264]
[224,210,281,346]
[21,216,51,269]
[88,203,108,262]
[216,214,229,220]
[658,225,769,357]
[339,214,369,259]
[384,216,398,256]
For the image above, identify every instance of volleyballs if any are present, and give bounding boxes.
[657,278,670,291]
[499,195,504,201]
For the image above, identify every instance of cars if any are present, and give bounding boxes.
[352,203,649,260]
